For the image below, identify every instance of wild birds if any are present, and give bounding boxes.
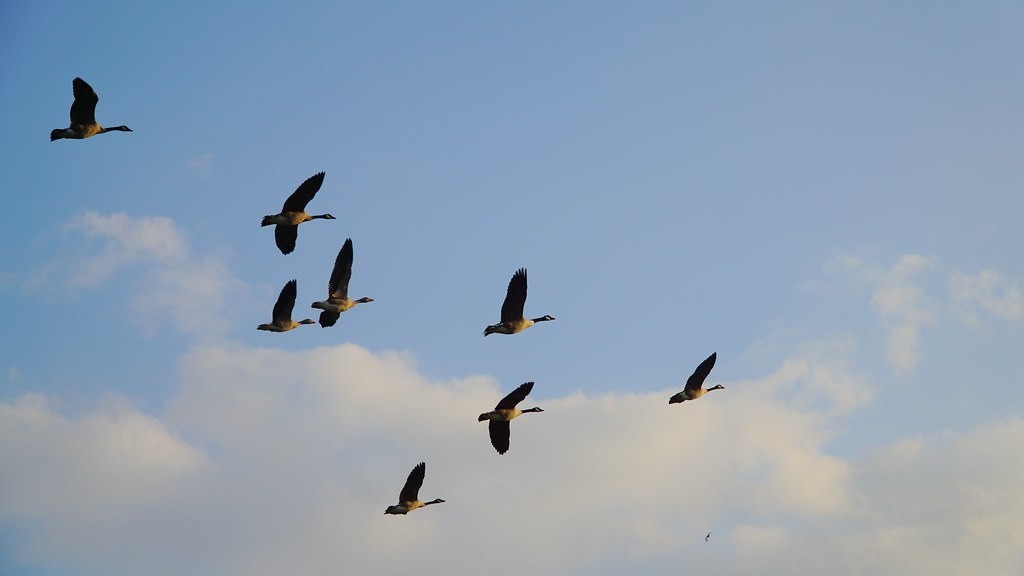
[477,382,544,455]
[261,172,336,255]
[50,77,133,142]
[310,237,374,328]
[482,267,555,337]
[384,462,446,515]
[668,352,725,405]
[256,279,315,332]
